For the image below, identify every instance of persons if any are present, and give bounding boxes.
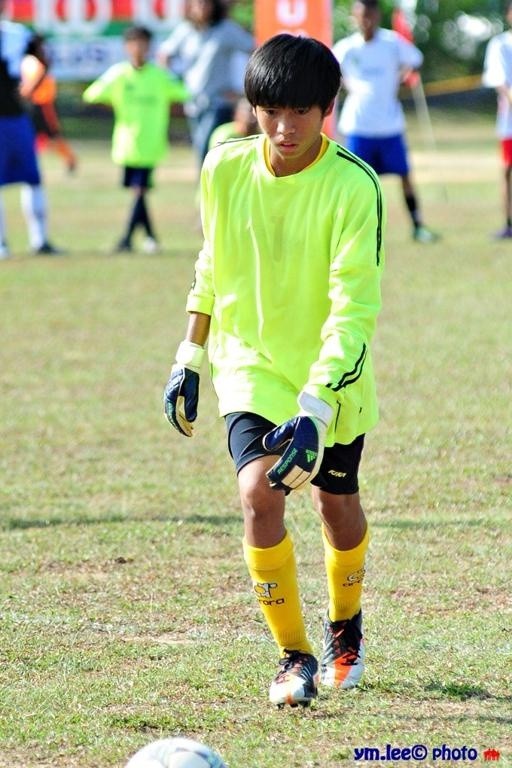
[80,27,192,255]
[163,33,383,709]
[0,50,62,258]
[330,1,441,246]
[156,0,261,170]
[481,0,512,241]
[17,37,78,174]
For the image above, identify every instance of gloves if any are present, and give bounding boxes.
[321,611,364,690]
[164,339,206,437]
[271,649,320,704]
[263,390,334,496]
